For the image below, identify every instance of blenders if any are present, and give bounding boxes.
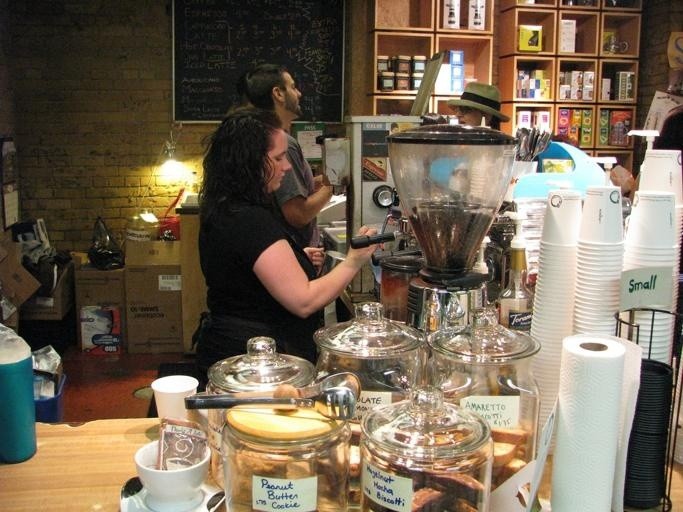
[384,126,518,329]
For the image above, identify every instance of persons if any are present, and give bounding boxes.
[447,80,511,130]
[197,104,380,395]
[243,62,335,275]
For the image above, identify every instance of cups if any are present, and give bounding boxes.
[311,250,347,278]
[149,374,198,422]
[135,441,212,512]
[518,149,683,456]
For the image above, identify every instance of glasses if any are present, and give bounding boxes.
[453,105,480,115]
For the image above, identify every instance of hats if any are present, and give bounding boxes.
[447,82,510,122]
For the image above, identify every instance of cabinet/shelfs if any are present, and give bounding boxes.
[366,1,495,117]
[495,0,643,175]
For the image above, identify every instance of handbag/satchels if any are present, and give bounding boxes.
[88,241,123,270]
[158,217,180,241]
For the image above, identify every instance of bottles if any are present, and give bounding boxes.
[205,300,544,512]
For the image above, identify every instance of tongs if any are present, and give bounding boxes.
[183,369,361,419]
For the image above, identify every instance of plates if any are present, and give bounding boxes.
[119,475,224,512]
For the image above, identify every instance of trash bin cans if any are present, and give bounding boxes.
[34,373,67,423]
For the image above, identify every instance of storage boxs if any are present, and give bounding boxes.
[0,184,73,422]
[124,240,182,355]
[73,252,128,355]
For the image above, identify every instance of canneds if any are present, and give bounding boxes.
[313,300,426,472]
[358,386,492,511]
[426,306,539,494]
[219,384,349,511]
[207,334,316,489]
